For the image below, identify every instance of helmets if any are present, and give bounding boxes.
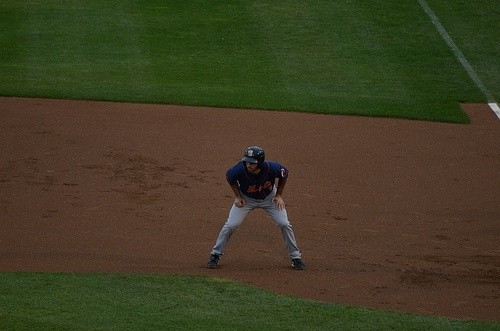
[242,145,265,163]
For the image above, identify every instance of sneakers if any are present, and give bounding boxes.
[291,258,306,270]
[207,253,220,269]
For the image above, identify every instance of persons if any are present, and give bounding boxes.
[207,145,306,270]
